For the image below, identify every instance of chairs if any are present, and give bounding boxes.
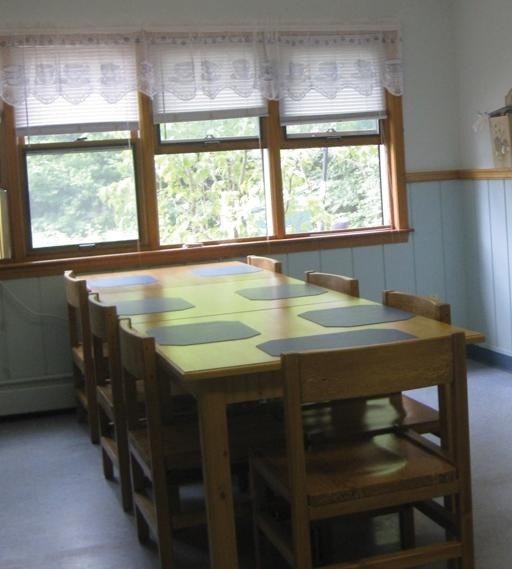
[66,256,483,567]
